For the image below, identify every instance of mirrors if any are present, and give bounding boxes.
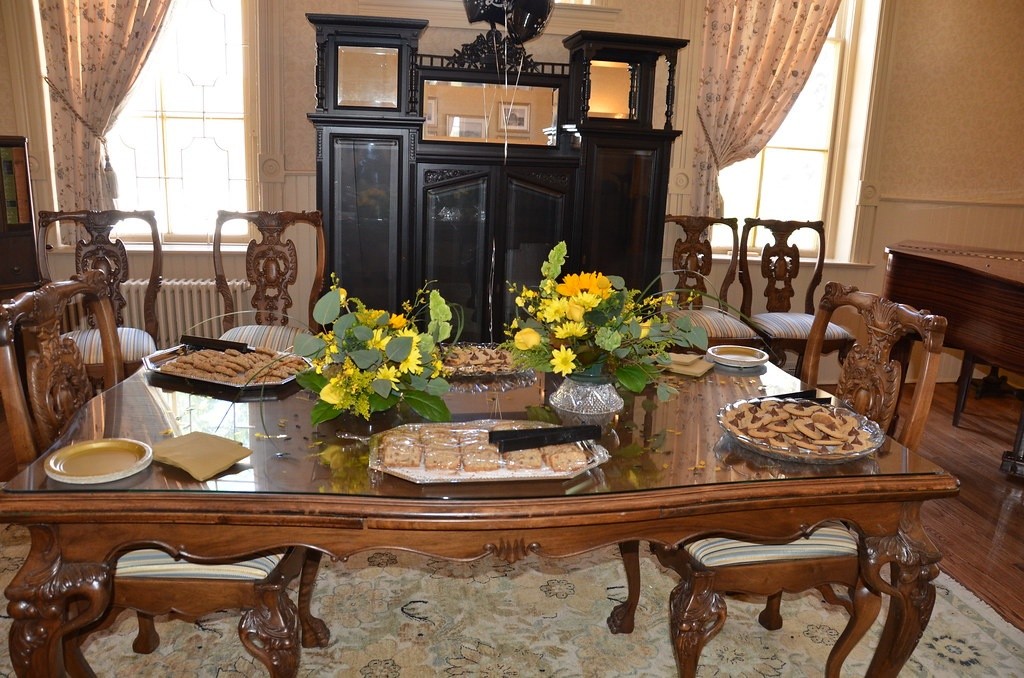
[422,79,559,146]
[337,46,399,108]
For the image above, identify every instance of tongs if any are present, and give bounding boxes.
[488,425,602,452]
[180,334,255,355]
[747,389,832,405]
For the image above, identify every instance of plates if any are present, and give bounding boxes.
[717,397,886,464]
[140,342,316,388]
[441,342,525,376]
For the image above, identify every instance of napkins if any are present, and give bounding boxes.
[651,351,703,366]
[656,359,716,377]
[152,431,254,482]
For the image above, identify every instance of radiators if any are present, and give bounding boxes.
[57,278,252,393]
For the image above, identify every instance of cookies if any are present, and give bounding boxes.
[160,346,307,384]
[721,400,873,459]
[439,343,517,372]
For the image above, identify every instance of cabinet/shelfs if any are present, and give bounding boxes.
[306,111,427,334]
[407,151,586,347]
[563,132,673,321]
[0,135,40,399]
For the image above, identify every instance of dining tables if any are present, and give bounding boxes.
[0,361,963,678]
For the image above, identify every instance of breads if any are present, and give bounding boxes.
[378,422,589,473]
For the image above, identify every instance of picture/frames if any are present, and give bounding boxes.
[445,113,490,139]
[497,101,531,134]
[427,96,439,128]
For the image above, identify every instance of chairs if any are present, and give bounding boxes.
[36,209,164,390]
[212,208,327,355]
[607,278,947,678]
[739,218,857,368]
[664,213,765,356]
[0,268,332,678]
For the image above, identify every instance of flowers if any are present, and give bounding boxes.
[496,241,779,403]
[181,271,465,452]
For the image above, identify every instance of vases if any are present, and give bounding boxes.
[547,375,625,432]
